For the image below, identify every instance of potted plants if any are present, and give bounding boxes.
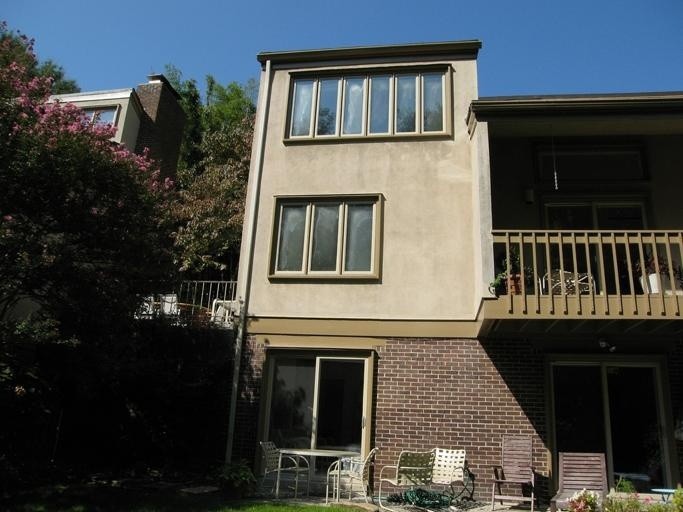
[208,462,258,500]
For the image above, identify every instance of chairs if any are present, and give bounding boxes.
[486,433,540,511]
[258,439,471,511]
